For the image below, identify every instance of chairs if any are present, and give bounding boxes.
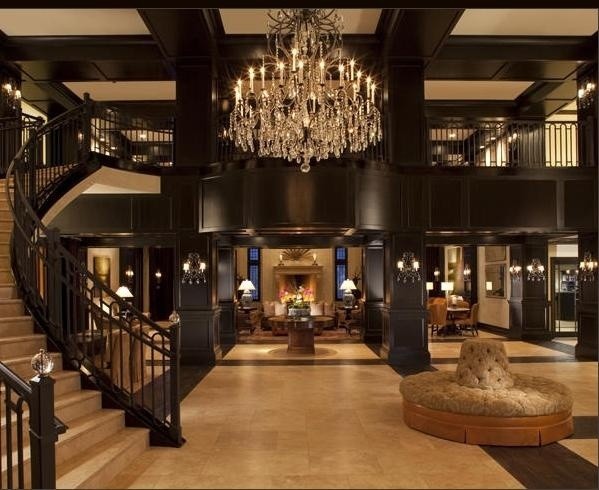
[428,298,480,339]
[341,298,366,336]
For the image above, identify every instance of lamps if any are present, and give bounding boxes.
[575,81,596,110]
[440,281,454,308]
[509,257,522,282]
[426,282,434,298]
[579,250,598,283]
[238,279,256,307]
[396,251,423,285]
[114,286,135,301]
[340,277,356,305]
[485,279,494,292]
[1,76,22,112]
[564,263,579,278]
[179,252,209,289]
[219,1,387,173]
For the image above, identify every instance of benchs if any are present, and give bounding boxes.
[391,336,579,449]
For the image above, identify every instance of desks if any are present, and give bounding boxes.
[286,327,316,355]
[238,309,259,333]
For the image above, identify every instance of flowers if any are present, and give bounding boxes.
[278,284,311,308]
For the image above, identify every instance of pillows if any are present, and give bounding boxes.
[309,301,324,316]
[274,303,287,315]
[323,301,336,317]
[262,302,275,317]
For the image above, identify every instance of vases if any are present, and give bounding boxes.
[288,307,312,316]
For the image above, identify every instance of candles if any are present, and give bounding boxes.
[279,253,282,260]
[313,253,317,261]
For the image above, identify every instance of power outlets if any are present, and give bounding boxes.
[527,257,546,282]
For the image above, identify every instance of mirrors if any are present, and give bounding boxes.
[483,263,509,299]
[550,257,584,337]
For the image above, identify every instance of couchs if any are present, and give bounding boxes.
[256,301,340,330]
